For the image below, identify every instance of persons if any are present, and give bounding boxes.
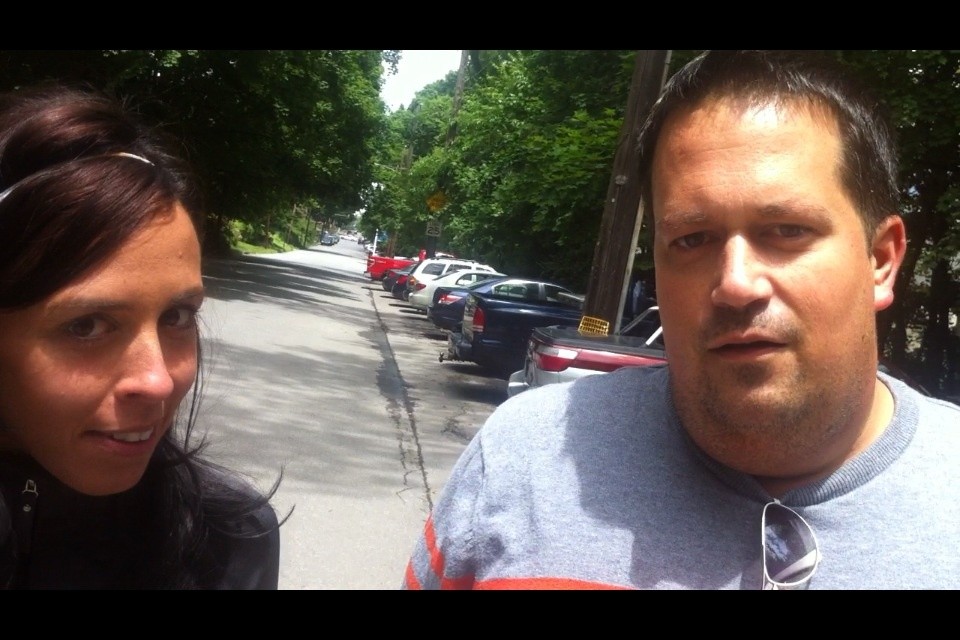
[399,50,960,590]
[0,83,293,591]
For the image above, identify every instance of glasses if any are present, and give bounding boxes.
[761,498,819,592]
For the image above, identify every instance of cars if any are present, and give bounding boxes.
[321,234,357,246]
[429,279,587,334]
[409,267,528,313]
[364,249,497,301]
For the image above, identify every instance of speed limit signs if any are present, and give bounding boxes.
[426,221,442,237]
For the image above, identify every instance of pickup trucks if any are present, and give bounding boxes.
[506,303,931,412]
[437,266,665,381]
